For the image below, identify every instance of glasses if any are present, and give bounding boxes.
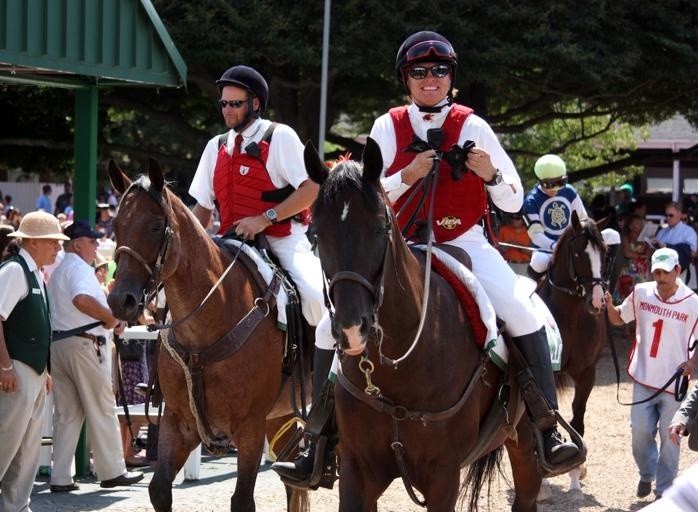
[536,175,569,190]
[663,212,675,219]
[617,217,624,223]
[509,212,524,221]
[219,99,249,109]
[406,64,451,80]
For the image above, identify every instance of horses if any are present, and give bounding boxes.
[538,209,612,504]
[303,136,570,512]
[107,157,313,512]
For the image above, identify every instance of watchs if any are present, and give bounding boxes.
[265,209,279,225]
[485,169,503,185]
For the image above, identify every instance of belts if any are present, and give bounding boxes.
[50,329,95,342]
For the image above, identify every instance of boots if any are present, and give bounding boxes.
[503,326,576,466]
[270,345,349,490]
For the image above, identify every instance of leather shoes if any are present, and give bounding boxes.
[133,384,153,400]
[635,480,652,500]
[48,482,81,496]
[99,470,144,487]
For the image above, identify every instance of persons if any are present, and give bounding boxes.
[603,247,698,497]
[191,65,326,373]
[92,250,110,286]
[270,32,588,488]
[0,173,170,222]
[0,212,70,512]
[613,185,640,213]
[669,381,698,452]
[615,217,651,302]
[494,209,539,280]
[648,205,698,289]
[47,223,141,490]
[522,154,609,314]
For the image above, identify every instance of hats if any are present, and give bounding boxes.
[94,252,112,272]
[650,249,679,275]
[614,183,636,204]
[0,224,14,238]
[63,219,107,243]
[7,211,71,242]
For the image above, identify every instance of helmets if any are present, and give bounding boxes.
[216,64,269,132]
[533,153,567,180]
[395,30,459,113]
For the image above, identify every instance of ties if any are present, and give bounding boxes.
[232,135,244,158]
